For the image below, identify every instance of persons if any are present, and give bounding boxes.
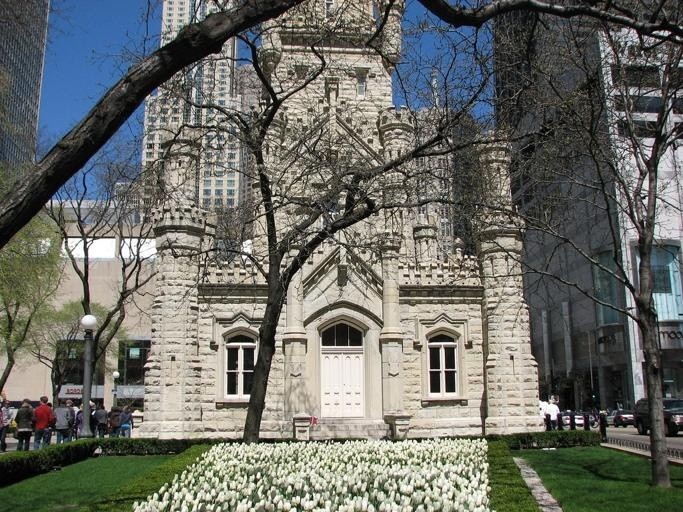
[592,407,598,421]
[0,396,134,451]
[541,394,560,429]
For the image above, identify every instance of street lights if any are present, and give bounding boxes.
[74,314,98,437]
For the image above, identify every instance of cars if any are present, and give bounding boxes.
[559,406,634,428]
[631,396,683,436]
